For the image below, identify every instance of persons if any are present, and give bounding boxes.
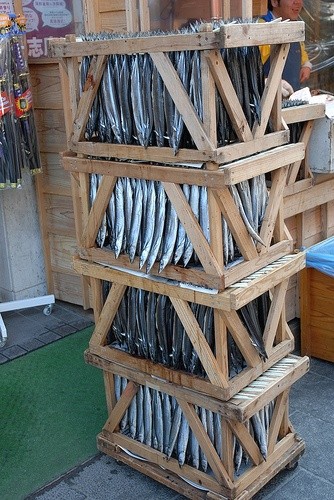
[252,0,312,98]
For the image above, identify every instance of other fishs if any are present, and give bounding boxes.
[71,14,286,475]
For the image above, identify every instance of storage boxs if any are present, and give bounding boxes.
[300,235,334,364]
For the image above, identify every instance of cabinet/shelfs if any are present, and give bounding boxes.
[47,21,309,500]
[281,95,334,323]
